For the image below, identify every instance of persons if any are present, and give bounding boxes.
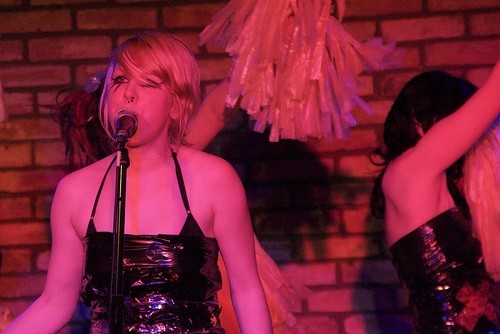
[368,61,499,334]
[4,33,274,334]
[60,60,318,333]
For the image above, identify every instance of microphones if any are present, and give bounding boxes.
[114,109,138,151]
